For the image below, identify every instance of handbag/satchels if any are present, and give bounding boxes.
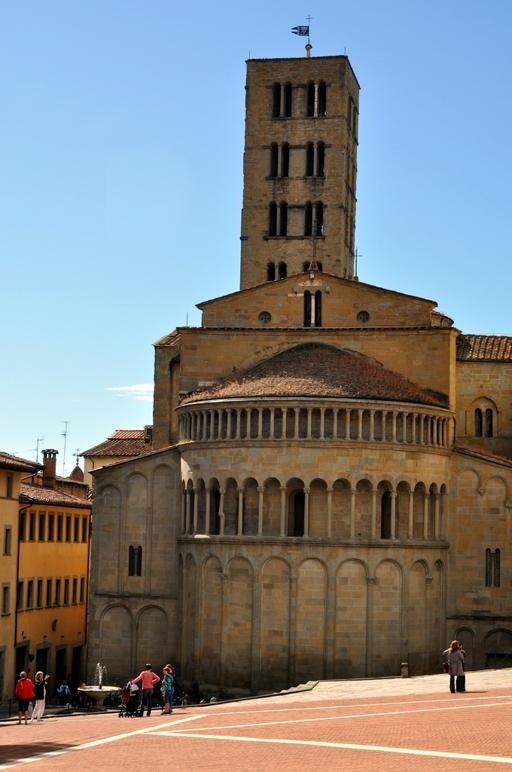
[442,663,448,673]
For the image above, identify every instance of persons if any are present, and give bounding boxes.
[442,640,467,693]
[129,662,176,717]
[15,670,87,725]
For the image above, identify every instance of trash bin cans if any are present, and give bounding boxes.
[400,662,409,677]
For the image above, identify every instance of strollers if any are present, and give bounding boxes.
[117,683,141,718]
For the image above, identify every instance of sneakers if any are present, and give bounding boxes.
[160,710,172,715]
